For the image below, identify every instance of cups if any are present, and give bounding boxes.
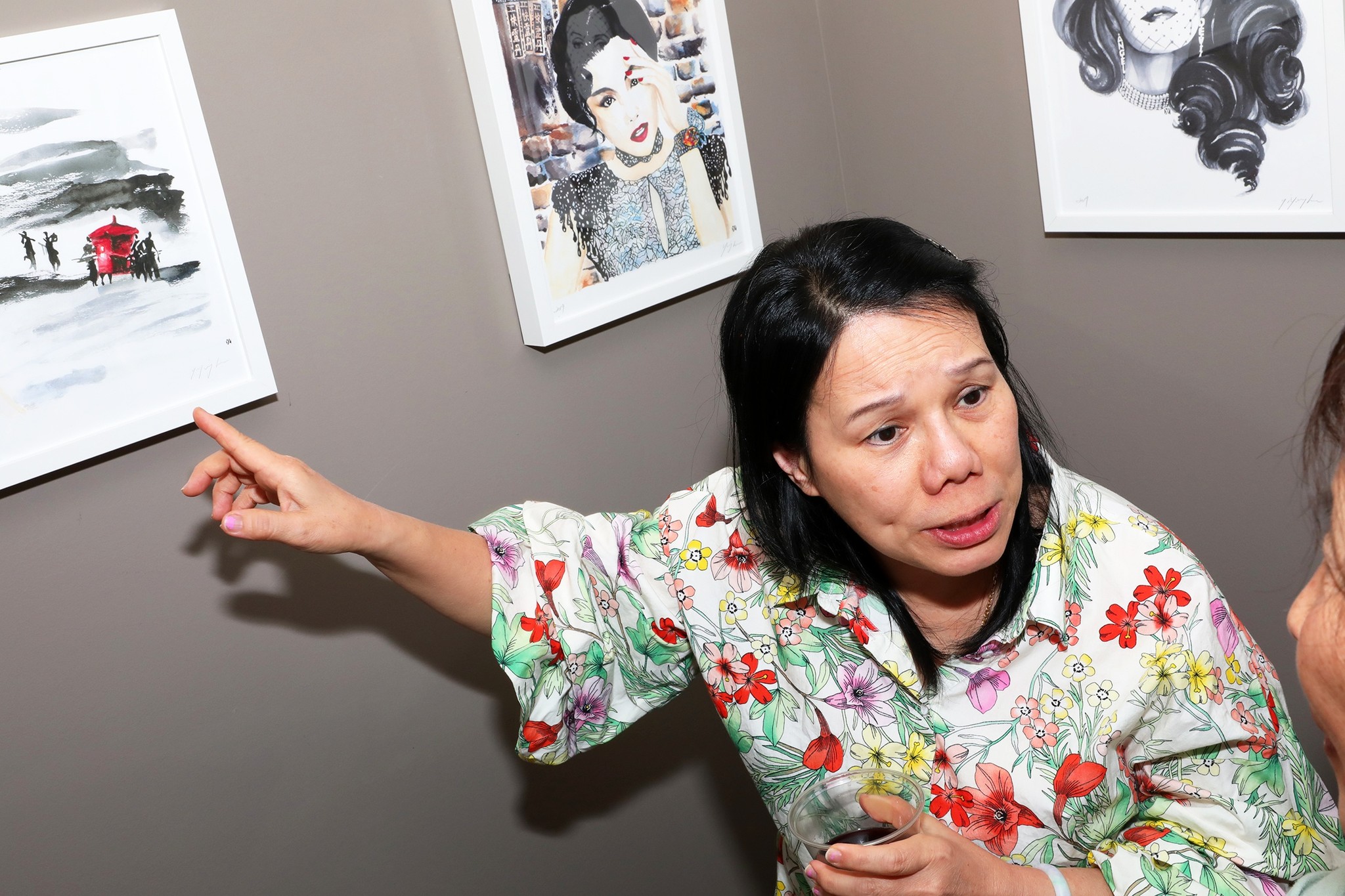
[785,765,924,866]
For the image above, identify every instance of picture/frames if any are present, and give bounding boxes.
[447,1,767,347]
[1015,0,1345,234]
[0,7,280,493]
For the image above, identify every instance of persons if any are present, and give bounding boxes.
[178,216,1345,896]
[1289,321,1345,838]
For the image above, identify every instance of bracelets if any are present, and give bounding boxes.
[1033,862,1075,896]
